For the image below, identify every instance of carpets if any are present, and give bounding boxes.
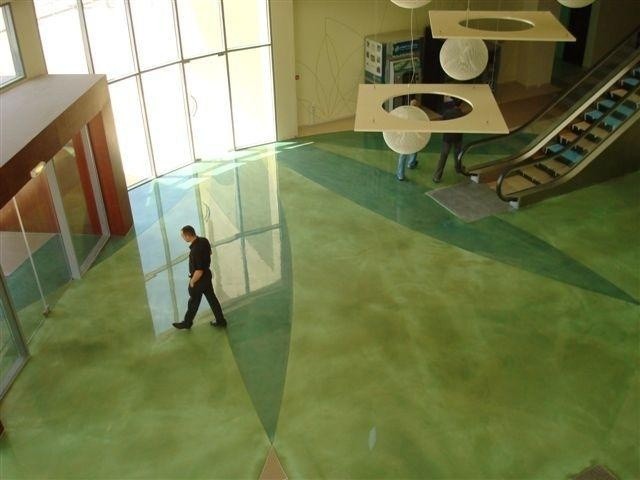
[425,181,519,226]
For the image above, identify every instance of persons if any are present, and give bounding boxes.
[395,99,421,181]
[432,99,466,182]
[172,226,228,329]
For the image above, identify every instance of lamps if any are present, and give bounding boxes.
[351,0,600,156]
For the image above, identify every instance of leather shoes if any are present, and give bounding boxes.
[209,320,226,327]
[432,176,440,184]
[172,322,191,329]
[396,175,405,181]
[407,160,419,168]
